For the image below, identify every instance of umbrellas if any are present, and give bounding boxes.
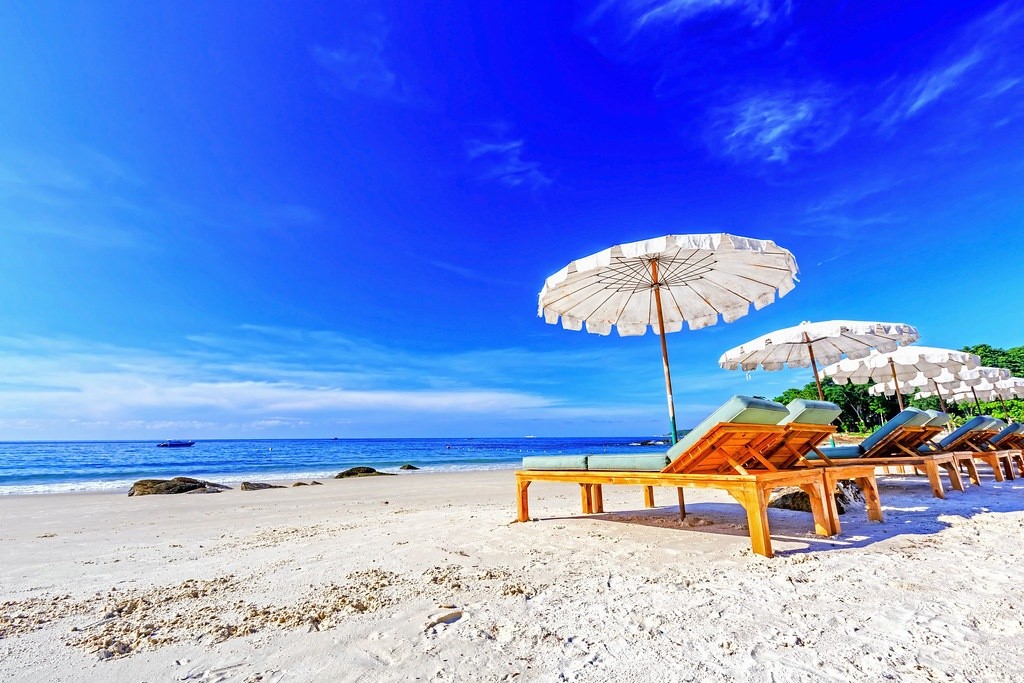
[817,345,980,474]
[535,232,800,520]
[717,319,922,450]
[869,367,1013,470]
[912,377,1024,479]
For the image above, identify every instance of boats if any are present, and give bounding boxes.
[157,439,196,447]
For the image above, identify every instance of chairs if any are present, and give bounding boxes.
[516,394,1024,558]
